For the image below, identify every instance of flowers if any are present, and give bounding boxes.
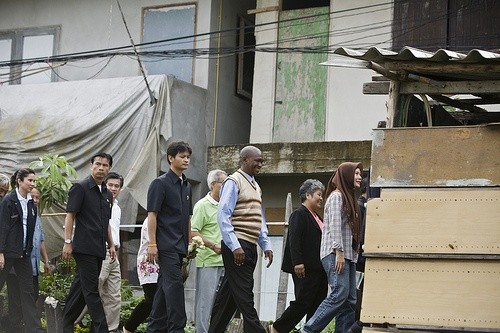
[182,236,205,267]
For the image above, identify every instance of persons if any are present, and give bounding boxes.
[208,146,273,333]
[0,151,163,333]
[267,161,374,333]
[147,141,192,333]
[190,169,230,333]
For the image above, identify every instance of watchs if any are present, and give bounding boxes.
[64,239,73,244]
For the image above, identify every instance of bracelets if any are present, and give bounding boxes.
[148,243,158,247]
[44,261,50,264]
[209,241,216,250]
